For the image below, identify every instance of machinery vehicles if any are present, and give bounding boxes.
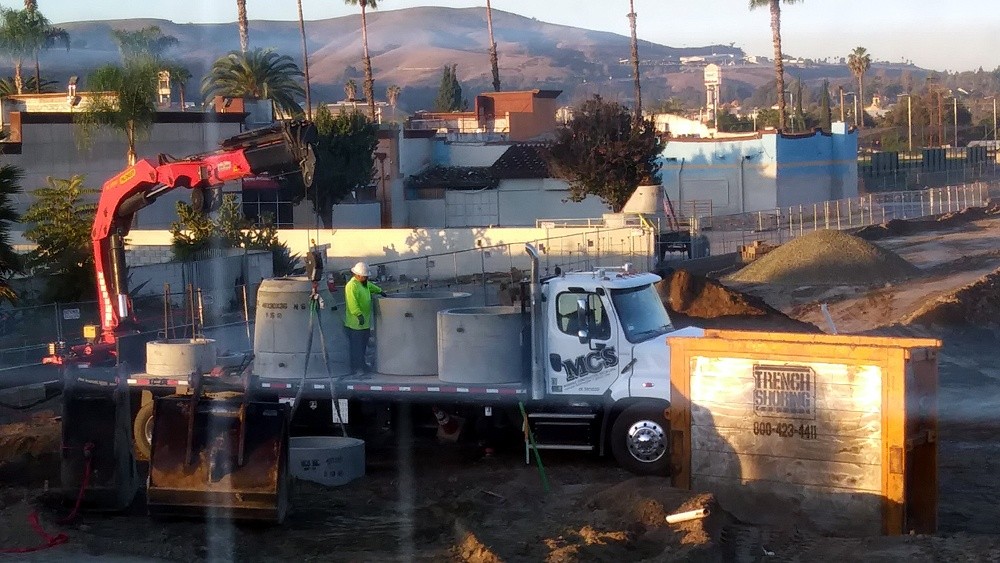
[654,191,711,262]
[41,119,320,370]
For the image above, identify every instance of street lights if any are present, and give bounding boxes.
[783,89,794,134]
[372,152,388,229]
[750,106,759,133]
[847,91,858,126]
[983,95,997,165]
[740,154,753,250]
[897,93,912,189]
[943,95,958,169]
[708,84,721,131]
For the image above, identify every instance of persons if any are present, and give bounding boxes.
[344,262,387,375]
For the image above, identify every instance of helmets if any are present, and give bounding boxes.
[351,262,372,277]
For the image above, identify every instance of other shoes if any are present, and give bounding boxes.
[351,371,369,379]
[365,366,377,374]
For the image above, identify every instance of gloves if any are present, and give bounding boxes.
[381,291,387,297]
[358,314,364,325]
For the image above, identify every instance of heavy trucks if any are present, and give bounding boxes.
[123,241,706,475]
